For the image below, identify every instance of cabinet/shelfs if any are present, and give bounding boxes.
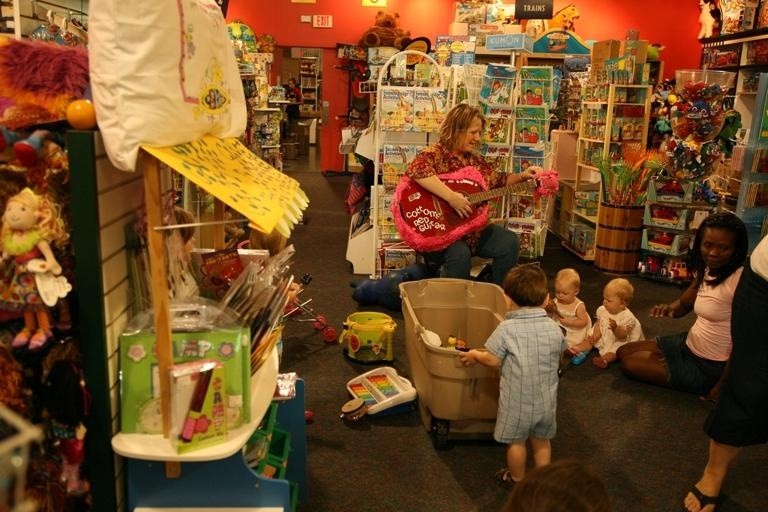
[370,50,563,280]
[698,26,768,255]
[109,150,313,512]
[299,57,318,112]
[560,83,655,261]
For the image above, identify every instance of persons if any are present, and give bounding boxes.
[0,190,70,352]
[685,229,768,512]
[459,264,566,490]
[546,269,593,356]
[287,79,303,122]
[502,461,610,512]
[617,213,757,400]
[569,279,645,369]
[42,336,91,492]
[403,105,542,289]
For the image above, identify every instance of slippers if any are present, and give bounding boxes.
[681,484,721,512]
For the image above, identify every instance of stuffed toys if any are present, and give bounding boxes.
[362,10,413,50]
[0,40,89,166]
[0,328,28,416]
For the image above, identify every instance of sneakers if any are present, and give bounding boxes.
[490,467,521,494]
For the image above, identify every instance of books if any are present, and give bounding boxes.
[378,63,552,273]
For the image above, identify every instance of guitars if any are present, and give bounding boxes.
[399,178,540,237]
